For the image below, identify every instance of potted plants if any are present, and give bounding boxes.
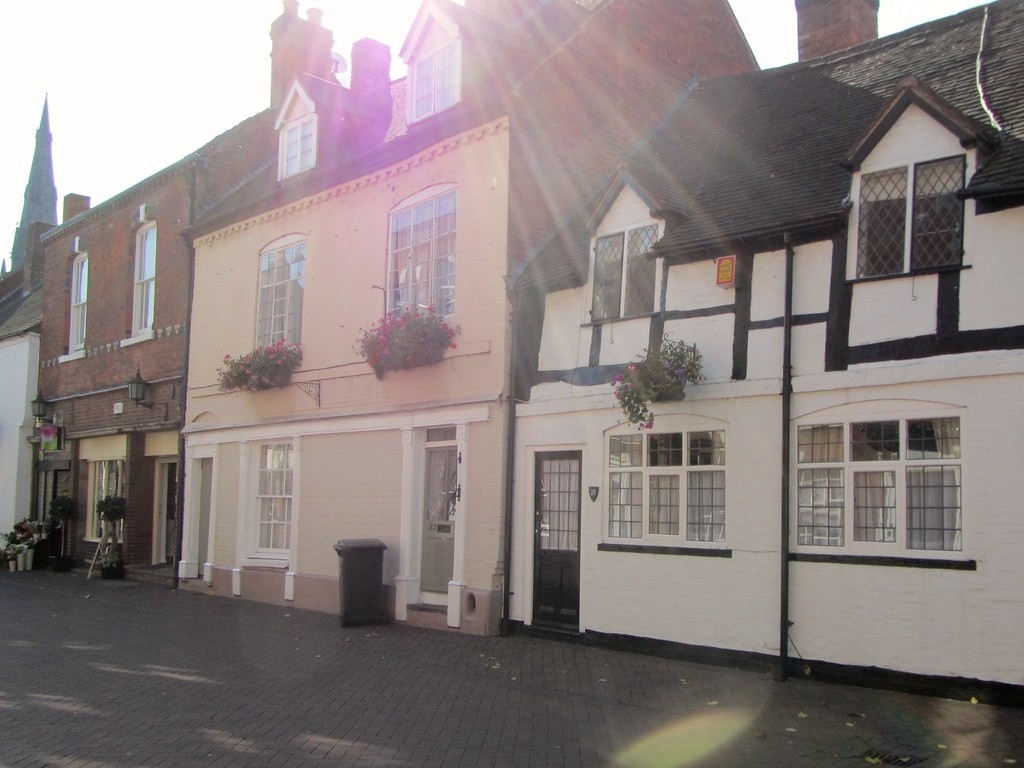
[42,525,48,539]
[34,524,40,542]
[96,494,126,579]
[51,496,77,572]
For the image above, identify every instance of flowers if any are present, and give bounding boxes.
[355,303,457,381]
[611,342,706,429]
[218,339,302,390]
[0,518,34,560]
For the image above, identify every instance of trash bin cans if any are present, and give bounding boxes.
[331,539,387,628]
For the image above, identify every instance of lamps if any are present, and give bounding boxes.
[31,394,46,416]
[129,368,146,400]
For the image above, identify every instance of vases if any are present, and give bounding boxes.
[18,555,24,571]
[26,549,33,570]
[10,561,16,571]
